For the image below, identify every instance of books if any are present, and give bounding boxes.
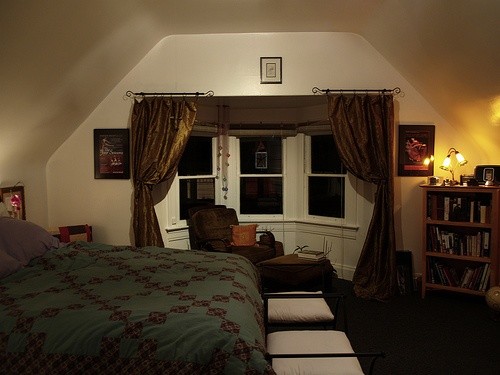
[427,194,490,224]
[297,250,324,260]
[427,227,490,259]
[425,261,490,291]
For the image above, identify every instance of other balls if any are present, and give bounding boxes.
[485,287,500,310]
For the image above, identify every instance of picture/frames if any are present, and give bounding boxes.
[0,186,26,219]
[260,57,282,84]
[94,129,130,180]
[397,124,435,176]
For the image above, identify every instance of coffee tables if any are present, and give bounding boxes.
[257,255,330,290]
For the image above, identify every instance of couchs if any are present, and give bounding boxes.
[188,205,275,264]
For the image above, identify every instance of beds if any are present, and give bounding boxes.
[0,243,275,375]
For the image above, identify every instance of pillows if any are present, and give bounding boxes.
[230,224,258,246]
[0,216,66,279]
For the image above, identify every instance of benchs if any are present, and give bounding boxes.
[266,329,365,375]
[263,289,341,331]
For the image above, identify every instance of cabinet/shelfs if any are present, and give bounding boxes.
[420,184,500,299]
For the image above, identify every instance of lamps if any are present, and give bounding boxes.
[440,148,466,185]
